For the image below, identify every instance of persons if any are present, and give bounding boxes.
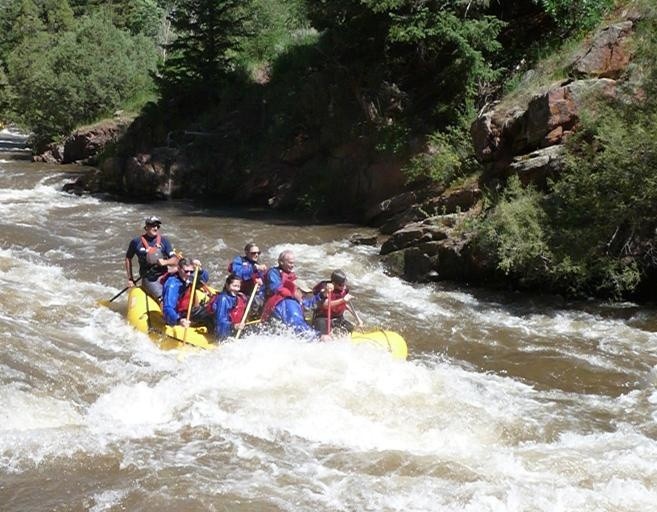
[208,275,267,342]
[263,249,297,307]
[125,216,178,310]
[314,269,358,341]
[228,242,268,296]
[162,256,214,334]
[260,278,335,342]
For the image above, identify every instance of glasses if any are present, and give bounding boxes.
[248,251,261,255]
[182,269,195,273]
[151,224,160,228]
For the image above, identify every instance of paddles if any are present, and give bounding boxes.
[176,264,202,363]
[96,264,158,309]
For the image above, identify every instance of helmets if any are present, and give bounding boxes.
[331,269,346,281]
[146,215,162,224]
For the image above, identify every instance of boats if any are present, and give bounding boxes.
[126,286,409,360]
